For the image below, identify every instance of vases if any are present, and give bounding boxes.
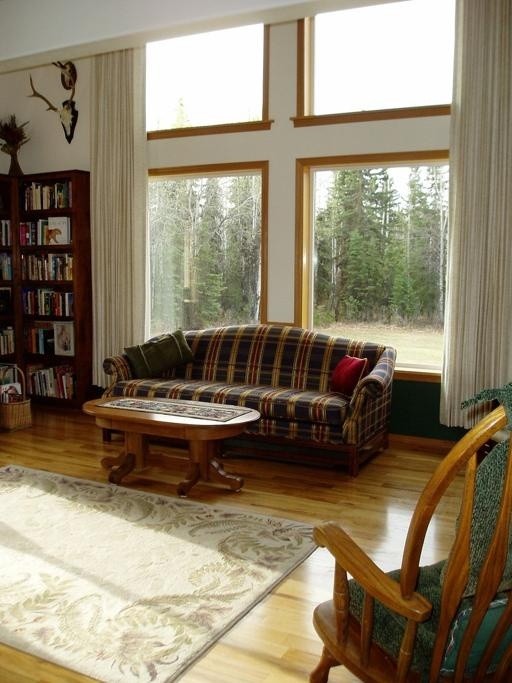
[9,153,23,176]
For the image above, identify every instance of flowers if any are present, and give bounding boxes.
[0,113,31,154]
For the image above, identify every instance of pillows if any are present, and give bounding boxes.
[442,599,512,674]
[123,326,195,378]
[330,354,368,400]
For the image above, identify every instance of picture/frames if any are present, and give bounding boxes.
[54,321,75,358]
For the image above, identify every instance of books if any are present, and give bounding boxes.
[0,181,74,404]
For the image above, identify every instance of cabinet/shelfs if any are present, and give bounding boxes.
[0,170,92,411]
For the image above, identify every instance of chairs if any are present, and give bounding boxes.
[310,404,512,683]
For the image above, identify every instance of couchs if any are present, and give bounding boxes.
[103,325,396,477]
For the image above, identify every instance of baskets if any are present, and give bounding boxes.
[0,366,32,432]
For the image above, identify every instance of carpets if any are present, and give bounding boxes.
[0,465,319,683]
